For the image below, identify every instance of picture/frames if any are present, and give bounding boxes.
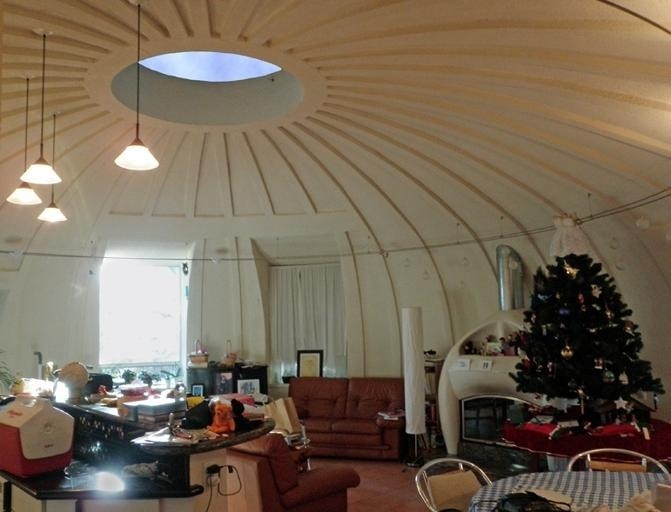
[191,384,204,397]
[296,349,324,377]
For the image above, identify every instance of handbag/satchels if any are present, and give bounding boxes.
[258,395,302,434]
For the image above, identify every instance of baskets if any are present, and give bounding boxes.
[224,340,237,367]
[189,339,209,363]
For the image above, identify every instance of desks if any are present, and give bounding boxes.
[470,470,671,512]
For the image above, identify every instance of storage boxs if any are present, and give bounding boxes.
[0,394,75,479]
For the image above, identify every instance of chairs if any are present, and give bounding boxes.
[566,449,667,472]
[414,457,494,511]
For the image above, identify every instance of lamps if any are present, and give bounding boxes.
[19,27,64,185]
[114,0,160,171]
[6,72,43,206]
[38,108,69,223]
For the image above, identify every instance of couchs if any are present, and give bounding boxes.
[287,377,403,462]
[189,432,362,511]
